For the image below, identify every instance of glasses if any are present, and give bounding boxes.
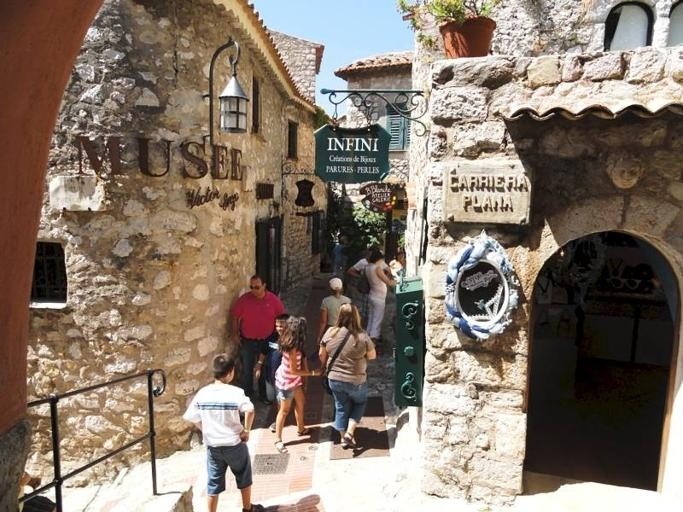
[250,286,260,289]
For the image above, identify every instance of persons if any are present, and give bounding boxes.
[252,314,289,432]
[181,353,265,512]
[331,234,406,358]
[314,277,351,397]
[316,302,376,450]
[230,274,284,404]
[274,314,321,456]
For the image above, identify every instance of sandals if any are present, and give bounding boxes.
[273,440,288,453]
[341,432,357,448]
[298,428,310,436]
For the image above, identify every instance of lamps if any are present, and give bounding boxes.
[203,37,252,149]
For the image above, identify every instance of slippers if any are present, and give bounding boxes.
[321,368,331,394]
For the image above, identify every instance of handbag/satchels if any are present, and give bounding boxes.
[357,275,369,293]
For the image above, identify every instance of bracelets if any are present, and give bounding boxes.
[257,359,264,366]
[312,369,315,377]
[243,428,249,434]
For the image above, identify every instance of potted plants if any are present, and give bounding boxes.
[399,1,501,59]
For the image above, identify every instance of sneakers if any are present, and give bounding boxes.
[270,422,276,432]
[243,504,264,512]
